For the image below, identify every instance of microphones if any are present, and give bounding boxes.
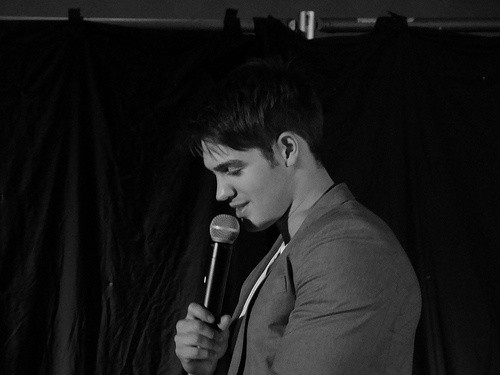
[203,214,239,332]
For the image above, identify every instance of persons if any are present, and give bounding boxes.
[176,60,424,372]
